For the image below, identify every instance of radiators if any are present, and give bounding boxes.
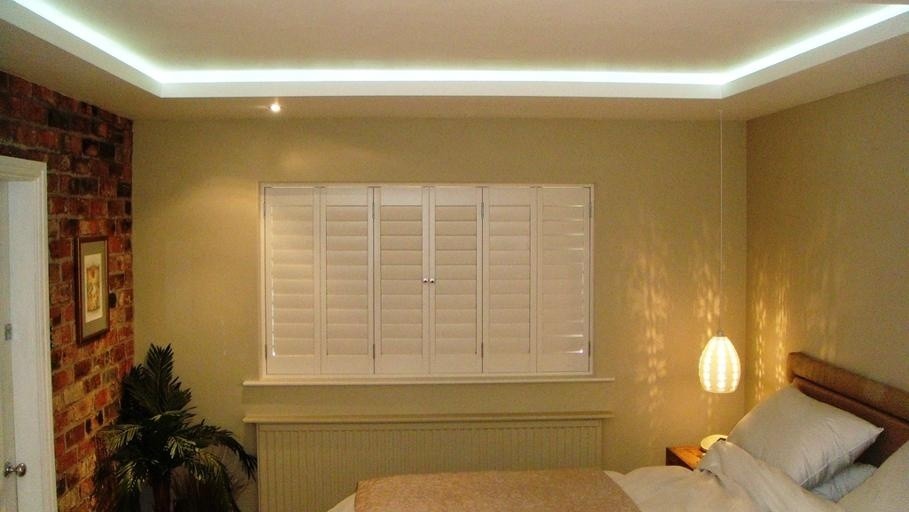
[242,411,616,512]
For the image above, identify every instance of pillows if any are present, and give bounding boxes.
[727,382,885,491]
[810,463,878,503]
[836,439,908,511]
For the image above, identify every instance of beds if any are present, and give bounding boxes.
[329,352,909,511]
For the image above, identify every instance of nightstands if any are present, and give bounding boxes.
[666,445,709,472]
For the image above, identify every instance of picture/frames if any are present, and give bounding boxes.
[74,236,110,346]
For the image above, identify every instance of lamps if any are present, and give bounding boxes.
[698,107,742,394]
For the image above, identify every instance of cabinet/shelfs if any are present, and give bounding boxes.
[259,184,596,381]
